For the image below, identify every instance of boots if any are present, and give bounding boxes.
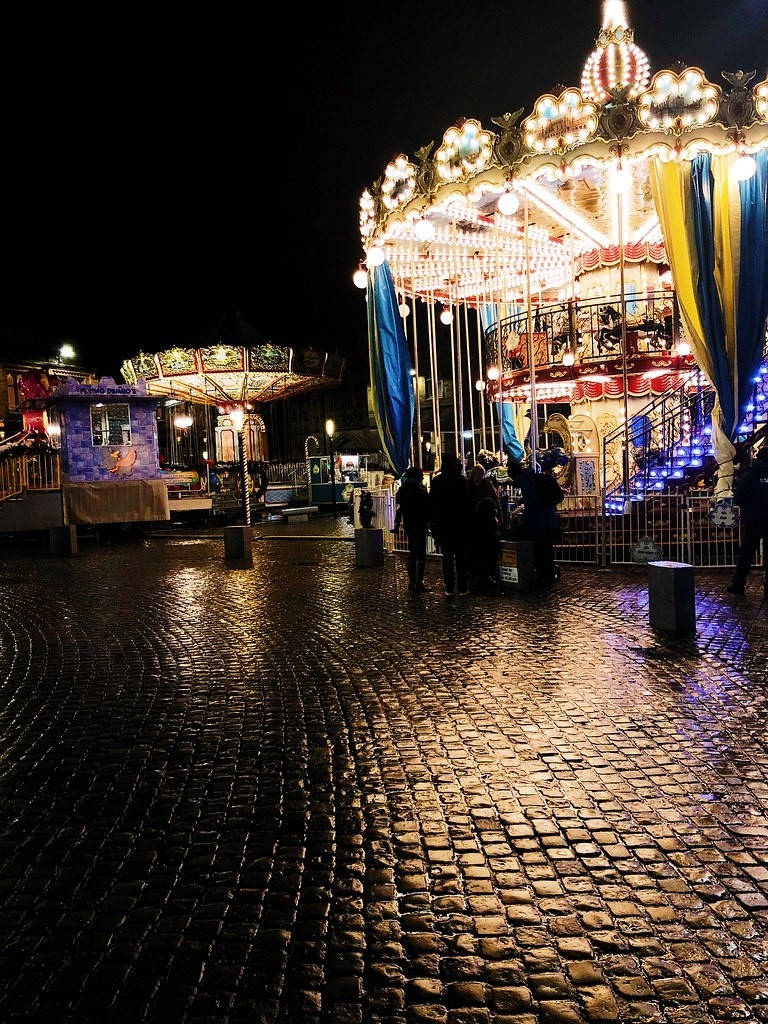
[415,583,432,592]
[408,578,416,590]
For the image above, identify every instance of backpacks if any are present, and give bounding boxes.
[524,472,563,507]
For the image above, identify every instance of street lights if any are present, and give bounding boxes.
[56,343,76,523]
[228,409,251,525]
[325,418,338,520]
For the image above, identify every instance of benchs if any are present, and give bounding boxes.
[270,506,318,524]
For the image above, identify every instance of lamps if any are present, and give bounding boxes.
[353,264,367,289]
[415,216,434,239]
[476,380,486,391]
[731,140,757,182]
[368,245,385,267]
[563,353,574,368]
[498,175,519,215]
[678,343,690,362]
[440,305,454,325]
[487,368,499,382]
[607,143,632,193]
[399,297,410,317]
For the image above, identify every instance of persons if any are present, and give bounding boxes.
[507,464,559,592]
[431,449,497,596]
[728,447,768,594]
[30,436,50,484]
[390,467,432,592]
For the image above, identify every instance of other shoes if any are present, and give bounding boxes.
[727,585,744,594]
[444,591,454,595]
[390,528,399,534]
[460,591,470,597]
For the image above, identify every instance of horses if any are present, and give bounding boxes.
[551,301,673,355]
[475,446,571,508]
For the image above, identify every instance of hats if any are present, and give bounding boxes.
[471,466,485,475]
